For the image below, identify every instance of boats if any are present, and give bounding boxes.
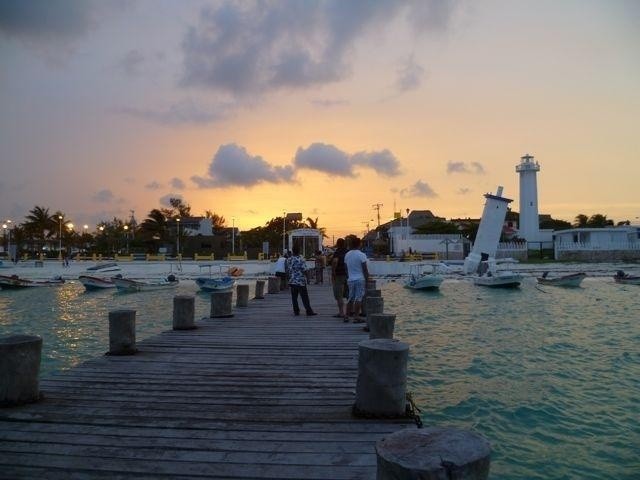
[537,271,587,288]
[404,262,444,292]
[614,271,640,285]
[472,258,524,288]
[4,260,179,293]
[195,264,237,291]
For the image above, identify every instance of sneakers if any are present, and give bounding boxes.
[332,310,366,325]
[306,312,319,317]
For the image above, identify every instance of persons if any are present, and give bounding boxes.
[324,248,328,255]
[286,246,312,312]
[287,251,293,266]
[333,236,346,317]
[316,250,325,284]
[276,253,289,289]
[344,238,370,319]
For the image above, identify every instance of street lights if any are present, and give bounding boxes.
[175,217,181,254]
[57,216,64,259]
[232,217,235,253]
[405,209,410,250]
[2,219,12,253]
[66,221,129,254]
[283,209,285,253]
